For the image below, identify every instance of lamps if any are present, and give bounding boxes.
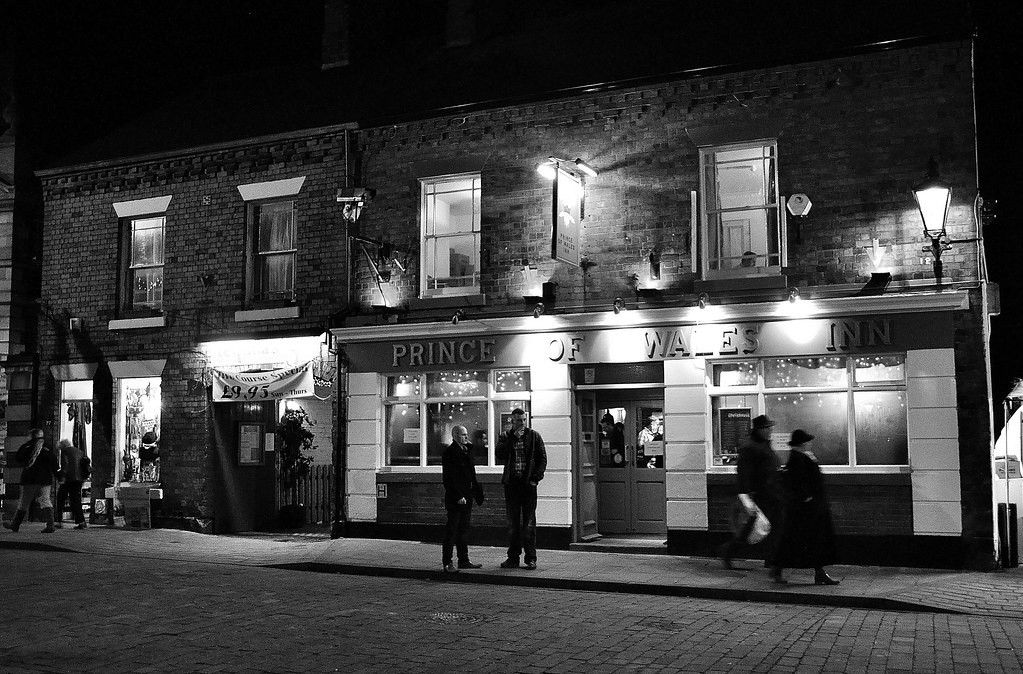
[639,288,662,301]
[371,304,391,313]
[522,295,543,304]
[910,158,953,261]
[871,272,891,283]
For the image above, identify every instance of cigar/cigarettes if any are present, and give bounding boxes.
[523,427,524,428]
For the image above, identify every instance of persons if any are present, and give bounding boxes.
[636,415,663,469]
[54,439,94,529]
[597,409,624,468]
[471,431,487,447]
[716,415,780,570]
[441,425,485,574]
[767,429,844,587]
[735,251,757,266]
[495,408,547,570]
[2,427,55,534]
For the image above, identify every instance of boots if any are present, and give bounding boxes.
[41,507,54,533]
[3,509,27,532]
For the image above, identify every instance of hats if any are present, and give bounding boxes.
[142,432,157,444]
[789,429,815,446]
[748,415,775,432]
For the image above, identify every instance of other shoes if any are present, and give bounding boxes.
[528,561,536,569]
[54,524,63,529]
[501,558,520,567]
[74,524,88,530]
[458,562,482,569]
[443,562,459,573]
[717,544,735,570]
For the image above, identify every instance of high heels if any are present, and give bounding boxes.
[769,571,788,584]
[815,571,841,585]
